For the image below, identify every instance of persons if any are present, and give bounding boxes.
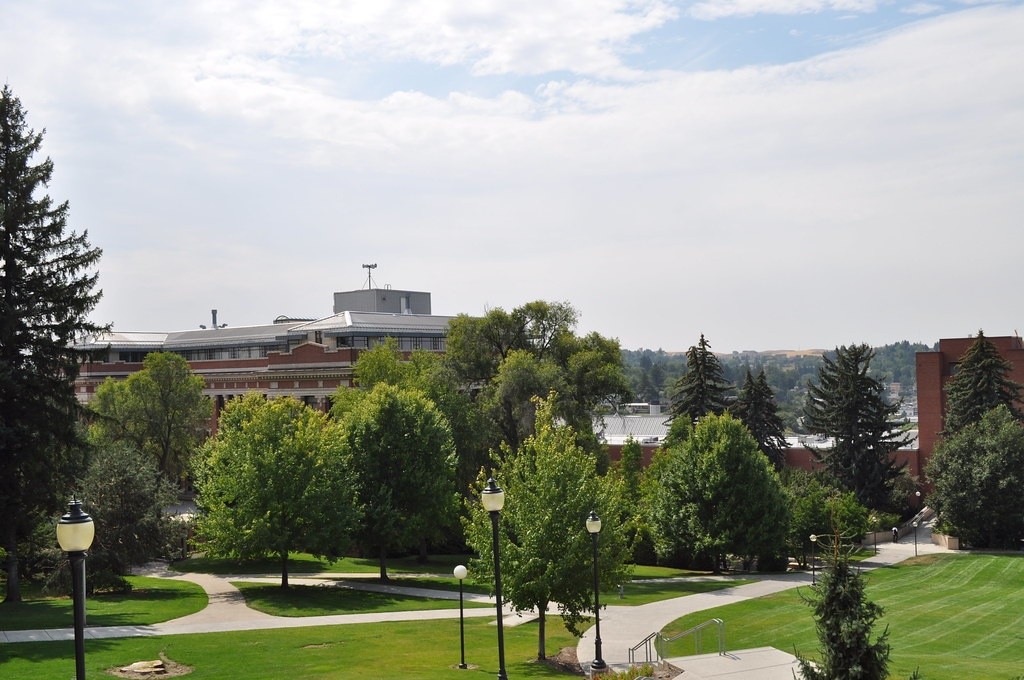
[892,525,898,542]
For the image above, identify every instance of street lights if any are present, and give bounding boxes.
[810,534,817,585]
[453,564,468,669]
[912,521,919,557]
[585,507,607,669]
[55,493,95,680]
[872,516,877,555]
[481,477,509,680]
